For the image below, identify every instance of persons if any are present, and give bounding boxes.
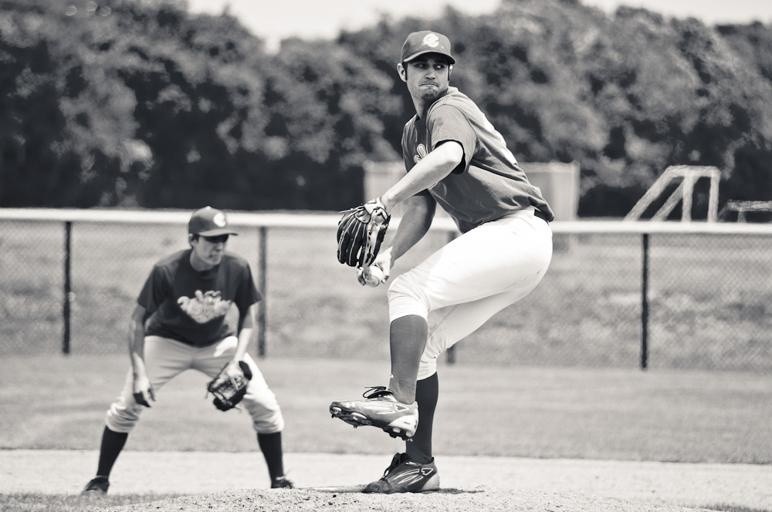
[77,204,294,492]
[329,30,555,496]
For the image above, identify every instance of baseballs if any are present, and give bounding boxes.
[364,268,382,287]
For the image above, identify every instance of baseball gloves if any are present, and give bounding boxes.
[208,362,253,412]
[335,199,390,268]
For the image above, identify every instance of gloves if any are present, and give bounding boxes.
[334,197,392,267]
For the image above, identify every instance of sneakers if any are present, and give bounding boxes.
[361,456,441,494]
[267,476,297,490]
[80,477,109,495]
[329,385,418,440]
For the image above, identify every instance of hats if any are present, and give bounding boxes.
[400,31,455,67]
[187,207,239,240]
